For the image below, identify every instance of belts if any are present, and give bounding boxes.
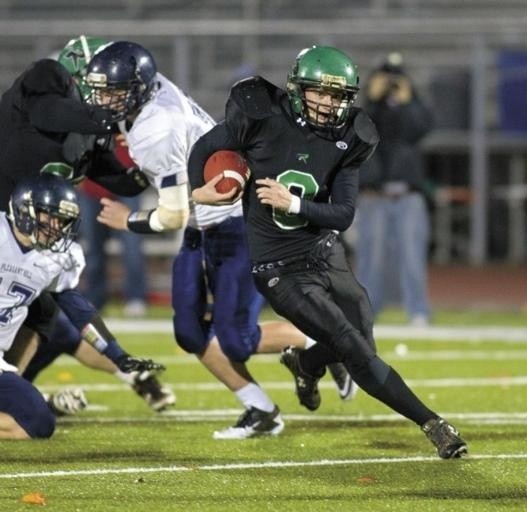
[249,229,341,275]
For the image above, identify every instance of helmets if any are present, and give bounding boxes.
[287,44,361,143]
[8,168,83,255]
[79,38,158,127]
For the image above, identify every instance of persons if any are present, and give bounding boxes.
[85,42,284,440]
[189,46,467,460]
[0,173,166,439]
[356,52,435,329]
[1,43,159,379]
[78,135,148,316]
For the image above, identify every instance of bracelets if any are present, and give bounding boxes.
[127,210,161,234]
[289,193,302,217]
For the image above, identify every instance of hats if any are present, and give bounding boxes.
[56,34,115,102]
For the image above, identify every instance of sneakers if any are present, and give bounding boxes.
[212,403,286,440]
[279,343,321,410]
[420,416,469,460]
[327,361,360,401]
[116,354,167,373]
[128,369,177,412]
[47,385,91,416]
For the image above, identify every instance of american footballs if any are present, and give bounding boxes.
[204,151,252,200]
